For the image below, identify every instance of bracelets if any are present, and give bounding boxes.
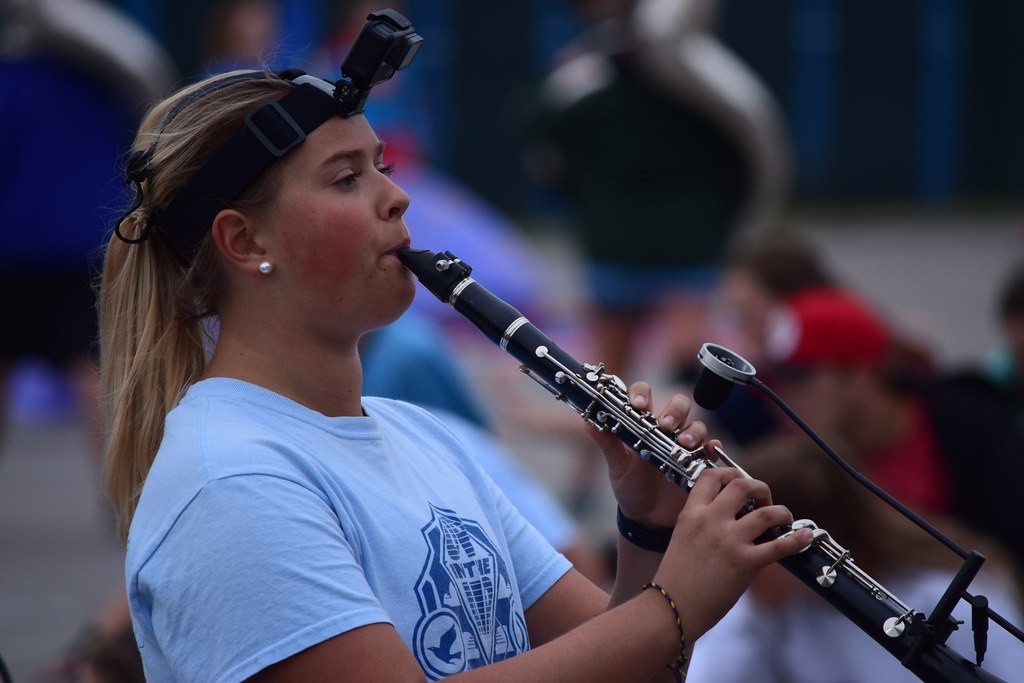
[616,506,678,551]
[643,582,689,683]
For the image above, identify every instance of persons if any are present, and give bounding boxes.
[0,0,1024,683]
[98,70,814,682]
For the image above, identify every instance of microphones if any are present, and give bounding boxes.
[693,343,756,411]
